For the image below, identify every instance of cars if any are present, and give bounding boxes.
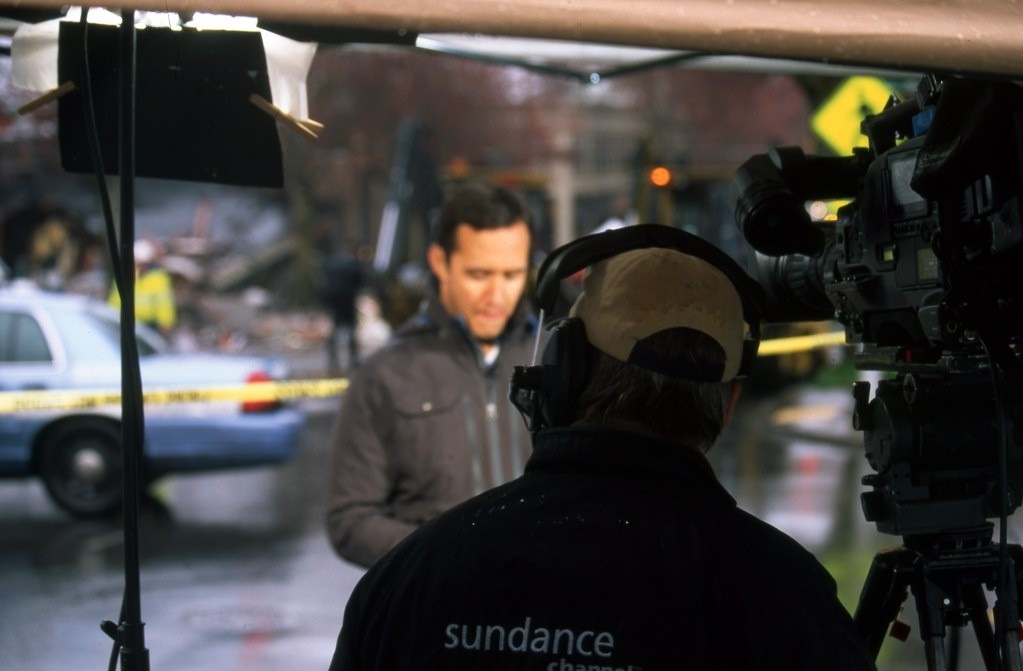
[0,280,306,518]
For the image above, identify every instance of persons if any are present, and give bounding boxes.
[326,186,556,569]
[107,239,177,336]
[328,247,880,671]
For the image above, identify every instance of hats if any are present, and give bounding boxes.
[566,246,745,385]
